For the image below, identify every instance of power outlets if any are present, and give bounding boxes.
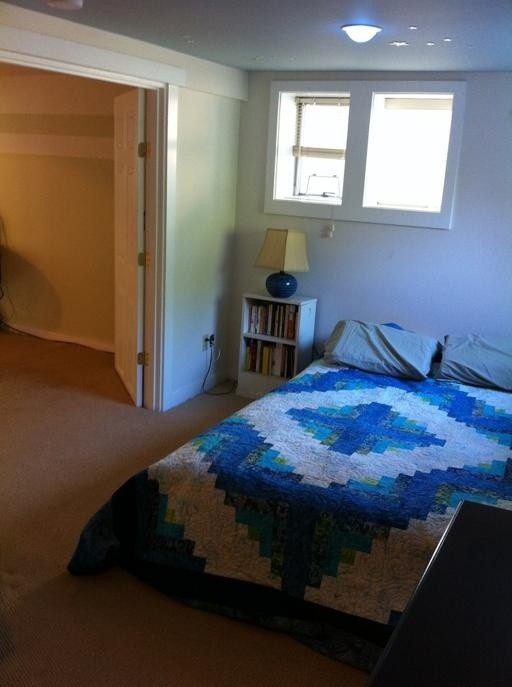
[201,334,208,351]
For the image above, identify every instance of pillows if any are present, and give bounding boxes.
[435,332,512,392]
[323,320,439,380]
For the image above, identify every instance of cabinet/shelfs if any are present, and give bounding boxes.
[235,294,317,400]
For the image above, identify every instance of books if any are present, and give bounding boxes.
[244,302,296,379]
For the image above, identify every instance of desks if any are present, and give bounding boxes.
[365,499,512,687]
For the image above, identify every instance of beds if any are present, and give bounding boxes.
[67,319,512,674]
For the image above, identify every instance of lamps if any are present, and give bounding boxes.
[253,227,310,298]
[341,23,383,44]
